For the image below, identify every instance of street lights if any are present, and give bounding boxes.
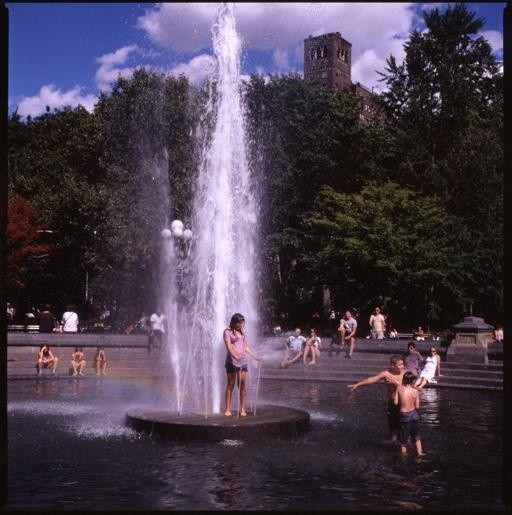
[160,218,194,285]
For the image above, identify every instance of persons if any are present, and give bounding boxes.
[346,355,407,441]
[301,328,323,365]
[334,304,504,359]
[7,299,171,376]
[277,326,308,370]
[222,313,264,418]
[403,342,424,381]
[412,345,446,389]
[393,372,427,457]
